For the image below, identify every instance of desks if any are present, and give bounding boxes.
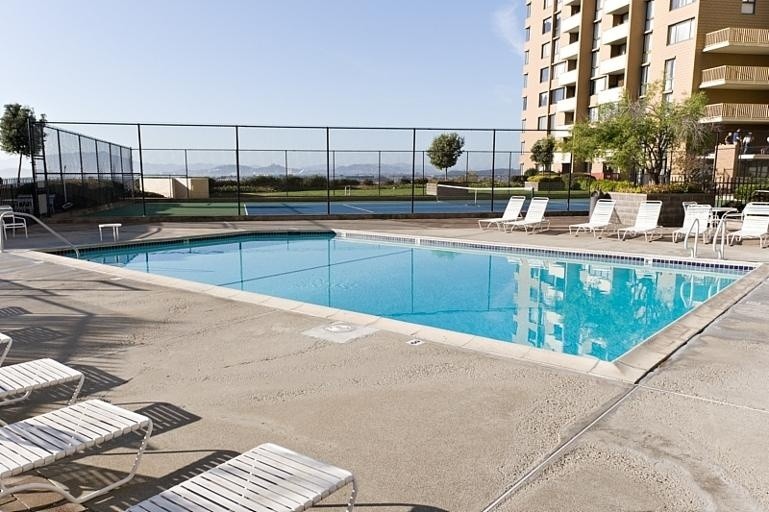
[98,223,123,241]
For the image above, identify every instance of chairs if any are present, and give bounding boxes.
[477,195,769,250]
[0,397,155,507]
[121,442,360,512]
[0,357,86,426]
[0,331,13,369]
[0,204,29,240]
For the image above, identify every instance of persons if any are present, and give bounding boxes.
[725,128,754,154]
